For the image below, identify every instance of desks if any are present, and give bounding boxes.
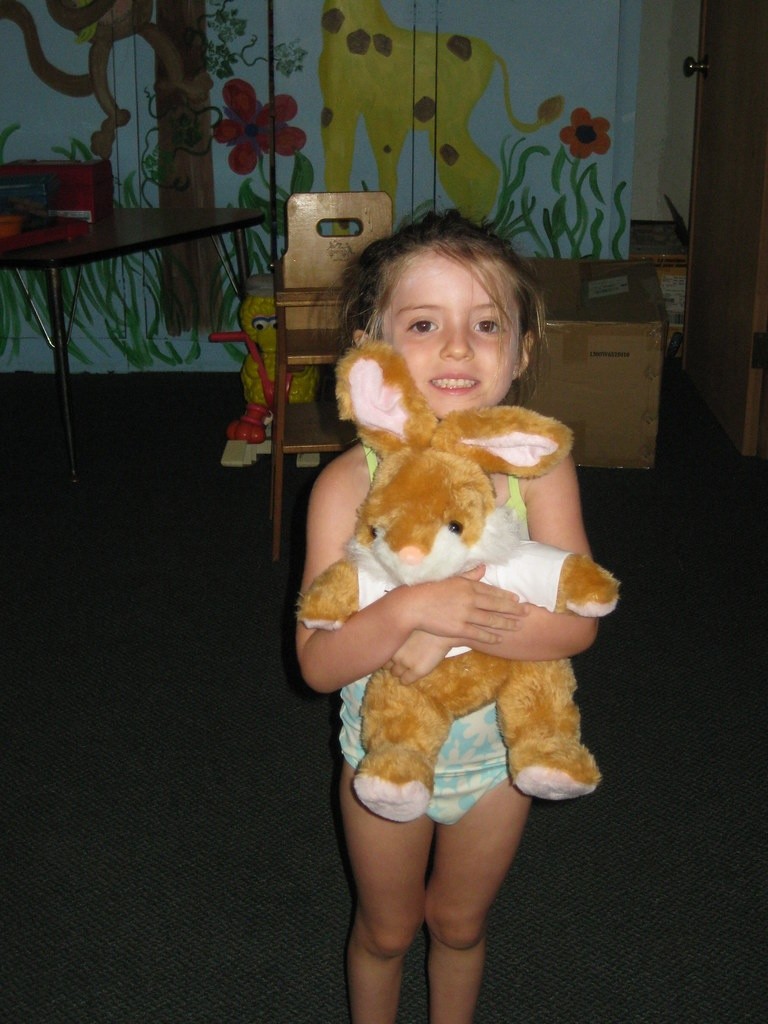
[0,207,265,482]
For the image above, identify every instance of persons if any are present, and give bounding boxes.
[295,208,597,1024]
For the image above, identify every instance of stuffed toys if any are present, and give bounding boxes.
[300,346,618,823]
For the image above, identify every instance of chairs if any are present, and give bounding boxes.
[268,192,393,561]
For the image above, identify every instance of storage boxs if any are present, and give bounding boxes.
[629,194,690,358]
[0,158,114,224]
[503,256,669,469]
